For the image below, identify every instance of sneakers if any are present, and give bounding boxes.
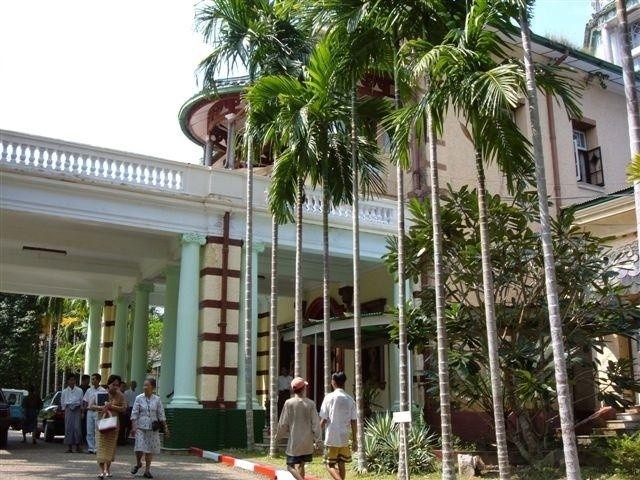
[96,470,113,479]
[131,462,143,475]
[144,472,153,479]
[21,437,37,444]
[64,448,97,454]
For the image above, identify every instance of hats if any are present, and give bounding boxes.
[290,376,309,391]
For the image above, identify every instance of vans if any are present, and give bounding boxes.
[0,387,28,433]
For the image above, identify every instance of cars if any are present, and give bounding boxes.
[0,390,10,448]
[38,392,86,443]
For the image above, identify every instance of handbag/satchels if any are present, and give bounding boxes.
[97,409,118,433]
[151,420,162,432]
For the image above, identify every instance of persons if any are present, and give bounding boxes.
[278,367,293,422]
[319,372,358,480]
[275,377,322,480]
[22,373,170,478]
